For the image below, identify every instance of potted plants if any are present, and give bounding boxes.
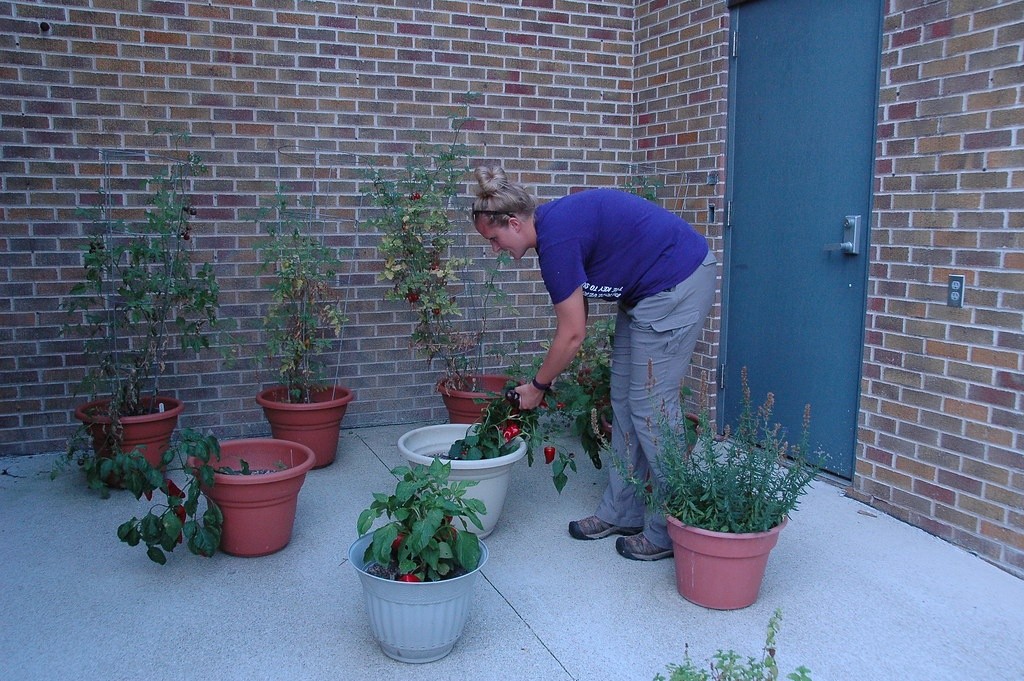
[67,114,828,666]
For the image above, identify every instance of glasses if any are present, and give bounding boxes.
[470,202,516,222]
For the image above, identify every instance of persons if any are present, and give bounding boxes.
[471,166,717,561]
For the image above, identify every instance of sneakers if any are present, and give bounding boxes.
[568,515,643,540]
[616,532,674,562]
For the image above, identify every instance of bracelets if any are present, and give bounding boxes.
[532,377,552,391]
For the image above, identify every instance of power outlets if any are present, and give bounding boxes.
[947,274,965,308]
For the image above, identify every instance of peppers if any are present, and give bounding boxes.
[391,514,457,582]
[494,406,555,464]
[143,478,207,557]
